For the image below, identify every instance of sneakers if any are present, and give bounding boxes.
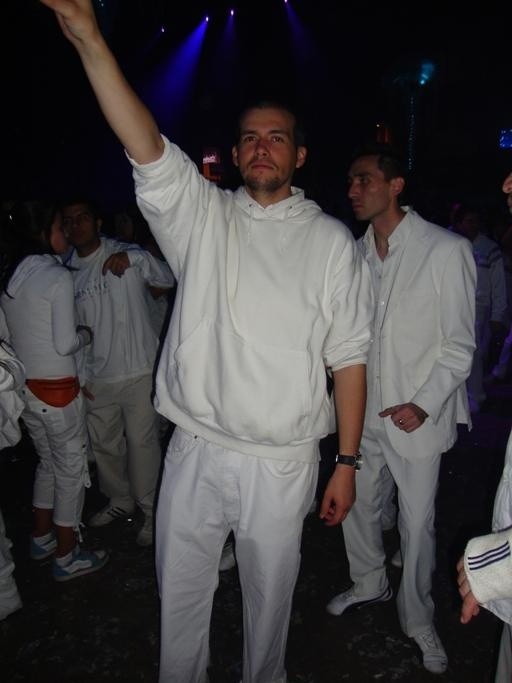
[89,498,138,527]
[410,626,448,674]
[31,537,59,560]
[136,516,156,546]
[327,584,392,616]
[54,550,109,583]
